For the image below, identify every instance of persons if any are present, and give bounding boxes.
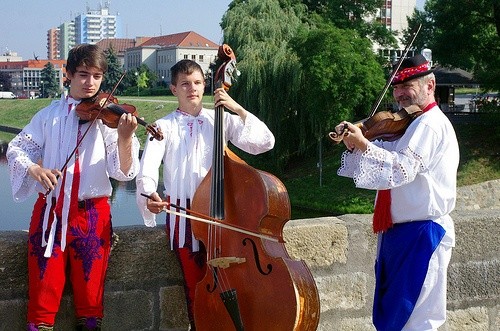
[335,55,460,331]
[135,59,276,331]
[5,44,141,331]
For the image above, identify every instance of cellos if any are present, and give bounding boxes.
[188,43,322,331]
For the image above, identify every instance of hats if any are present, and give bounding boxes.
[386,55,436,86]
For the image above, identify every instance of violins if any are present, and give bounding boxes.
[328,104,424,144]
[75,93,166,142]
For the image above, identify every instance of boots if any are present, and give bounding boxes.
[78,317,102,331]
[27,323,53,331]
